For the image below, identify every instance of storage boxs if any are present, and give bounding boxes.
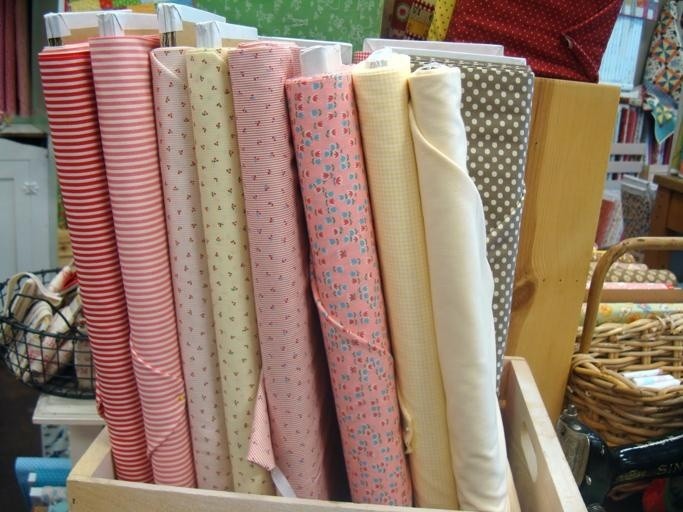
[67,355,588,511]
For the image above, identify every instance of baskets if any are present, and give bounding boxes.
[0,268,99,399]
[565,237,683,446]
